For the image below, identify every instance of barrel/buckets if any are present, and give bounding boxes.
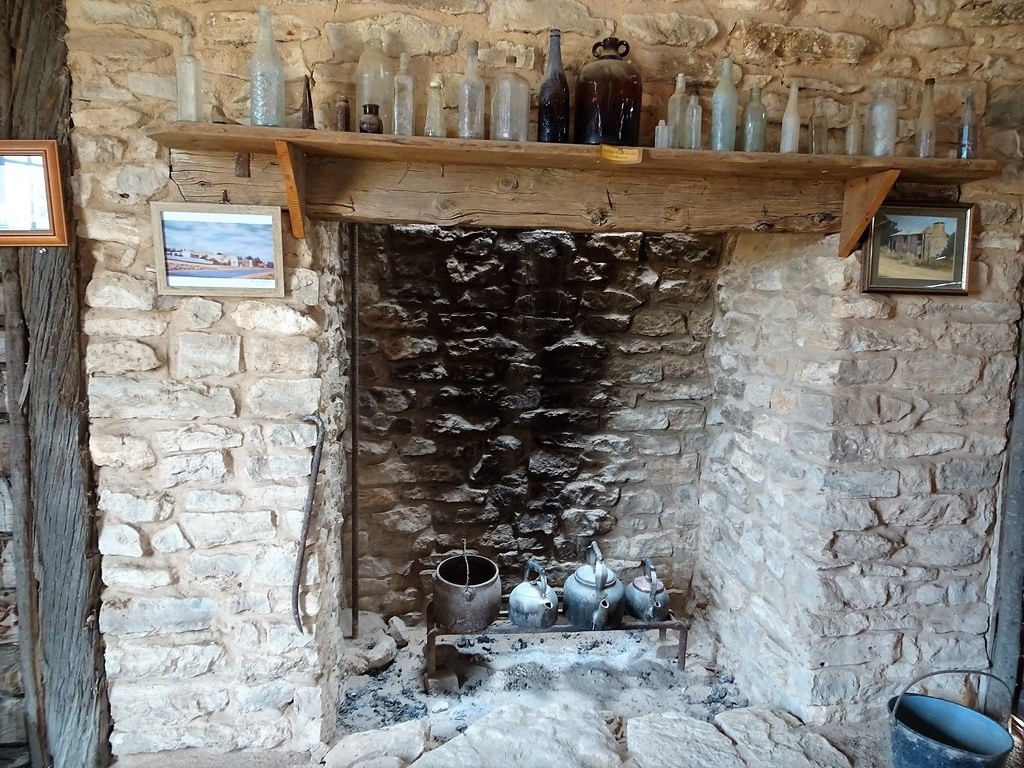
[885,667,1014,768]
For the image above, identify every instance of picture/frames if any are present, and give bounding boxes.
[859,202,976,296]
[0,140,70,247]
[151,201,286,297]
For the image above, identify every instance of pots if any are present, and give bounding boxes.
[431,553,503,633]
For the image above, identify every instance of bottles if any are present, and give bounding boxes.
[538,29,570,145]
[392,51,415,138]
[956,83,978,159]
[864,85,898,156]
[740,84,769,151]
[175,32,201,122]
[780,74,801,155]
[456,38,486,140]
[845,100,863,155]
[710,57,738,151]
[915,77,937,158]
[249,5,286,128]
[654,71,703,150]
[358,103,383,132]
[489,52,532,142]
[354,37,391,133]
[423,78,447,137]
[335,94,351,131]
[574,36,643,144]
[807,96,829,155]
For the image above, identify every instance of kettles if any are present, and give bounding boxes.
[505,559,559,630]
[563,538,624,628]
[626,557,673,624]
